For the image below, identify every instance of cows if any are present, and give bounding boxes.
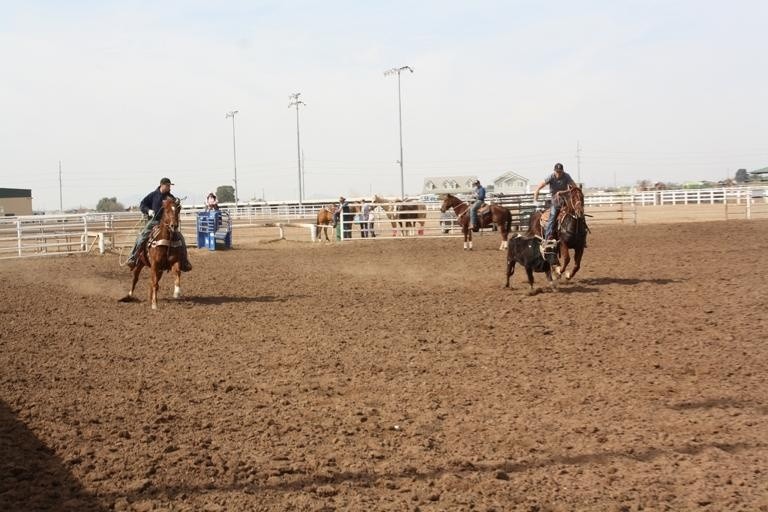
[506,233,561,292]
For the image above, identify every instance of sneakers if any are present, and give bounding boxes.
[468,224,478,230]
[127,254,136,268]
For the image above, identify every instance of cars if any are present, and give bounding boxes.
[437,209,461,233]
[418,194,436,202]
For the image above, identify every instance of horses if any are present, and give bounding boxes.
[315,200,361,243]
[440,193,512,251]
[125,197,187,310]
[370,195,426,237]
[525,183,588,281]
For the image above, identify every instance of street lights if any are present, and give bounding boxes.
[381,64,414,202]
[287,90,308,215]
[224,109,240,219]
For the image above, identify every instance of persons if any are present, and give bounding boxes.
[204,192,223,225]
[128,177,192,272]
[468,180,485,232]
[358,199,369,238]
[534,163,576,239]
[332,197,349,238]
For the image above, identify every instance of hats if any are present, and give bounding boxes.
[207,193,216,201]
[553,163,563,172]
[160,178,174,185]
